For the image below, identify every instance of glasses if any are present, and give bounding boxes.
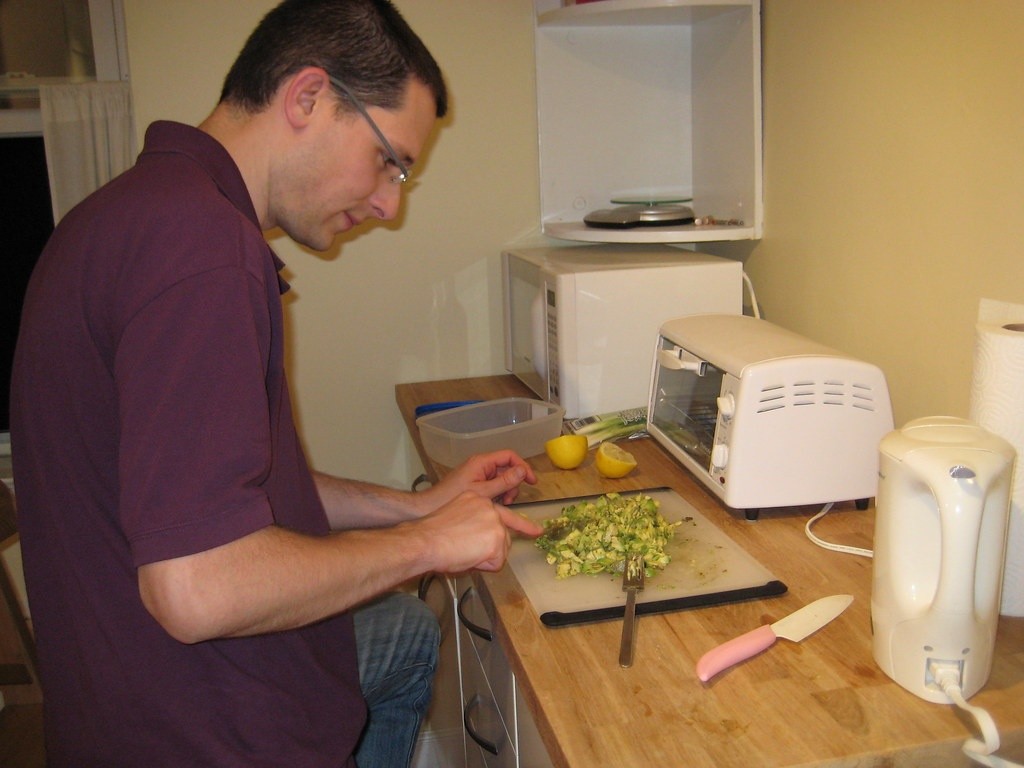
[328,77,415,186]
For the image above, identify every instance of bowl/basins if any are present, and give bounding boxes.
[415,396,566,469]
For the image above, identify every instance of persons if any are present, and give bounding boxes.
[10,0,545,768]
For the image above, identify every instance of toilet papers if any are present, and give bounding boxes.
[968,295,1024,618]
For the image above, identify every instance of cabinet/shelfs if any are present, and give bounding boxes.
[401,470,550,768]
[532,0,764,242]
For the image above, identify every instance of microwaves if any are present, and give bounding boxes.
[503,244,745,419]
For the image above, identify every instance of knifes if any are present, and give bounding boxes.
[694,594,855,682]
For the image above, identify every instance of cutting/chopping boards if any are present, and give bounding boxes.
[501,486,788,629]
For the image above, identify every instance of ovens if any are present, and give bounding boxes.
[644,313,896,520]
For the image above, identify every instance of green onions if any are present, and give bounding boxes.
[575,414,646,446]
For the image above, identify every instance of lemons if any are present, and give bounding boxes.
[596,442,636,478]
[545,435,588,470]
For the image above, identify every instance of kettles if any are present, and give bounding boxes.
[869,413,1018,708]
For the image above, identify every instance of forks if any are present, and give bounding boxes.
[618,553,645,668]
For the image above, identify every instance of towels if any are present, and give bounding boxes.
[36,83,138,226]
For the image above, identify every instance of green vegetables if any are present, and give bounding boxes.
[536,492,682,577]
